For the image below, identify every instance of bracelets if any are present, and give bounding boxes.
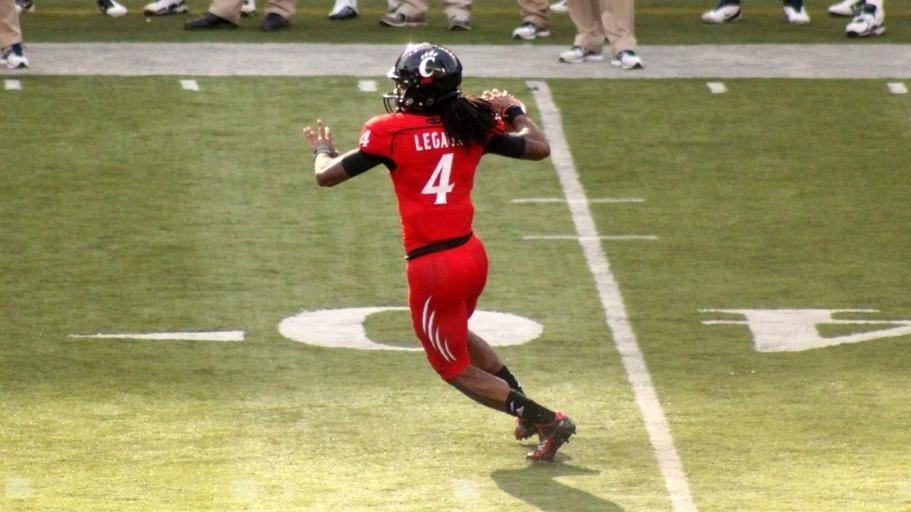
[500,103,525,127]
[312,146,332,161]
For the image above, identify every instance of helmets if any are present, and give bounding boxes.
[386,43,463,110]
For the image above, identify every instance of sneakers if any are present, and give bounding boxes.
[514,416,577,461]
[0,43,29,72]
[15,2,35,14]
[240,0,257,16]
[610,50,645,72]
[143,0,187,17]
[261,14,288,32]
[448,15,471,31]
[327,5,354,19]
[827,0,886,38]
[782,4,811,26]
[700,3,742,25]
[558,46,605,66]
[98,1,128,18]
[184,11,235,32]
[512,22,551,41]
[381,12,425,28]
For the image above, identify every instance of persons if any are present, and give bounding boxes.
[302,44,576,458]
[1,1,886,74]
